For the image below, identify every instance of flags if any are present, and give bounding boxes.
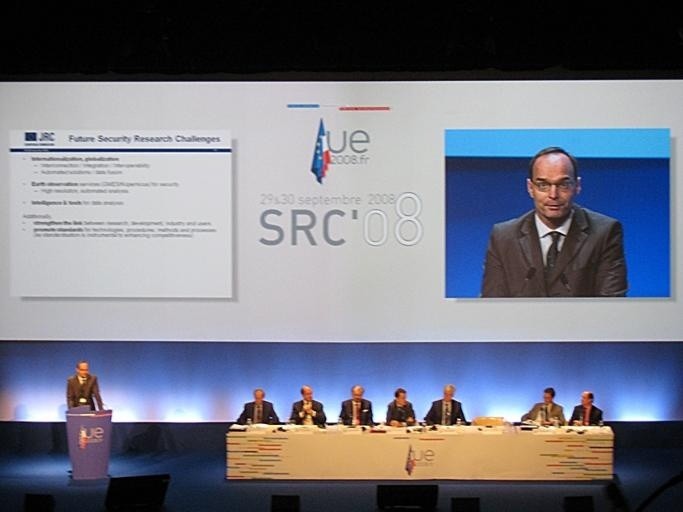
[405,444,416,476]
[311,118,331,184]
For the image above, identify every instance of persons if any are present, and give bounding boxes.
[481,147,627,297]
[568,392,602,426]
[521,388,565,426]
[339,386,374,425]
[386,388,415,427]
[422,385,466,426]
[290,386,326,425]
[238,389,278,425]
[67,361,104,411]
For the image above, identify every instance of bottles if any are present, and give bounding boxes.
[552,416,559,430]
[245,419,252,432]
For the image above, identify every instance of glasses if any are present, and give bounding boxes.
[531,180,574,192]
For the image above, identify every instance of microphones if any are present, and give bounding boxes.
[560,273,572,296]
[520,267,537,297]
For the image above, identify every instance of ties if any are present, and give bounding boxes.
[546,232,560,268]
